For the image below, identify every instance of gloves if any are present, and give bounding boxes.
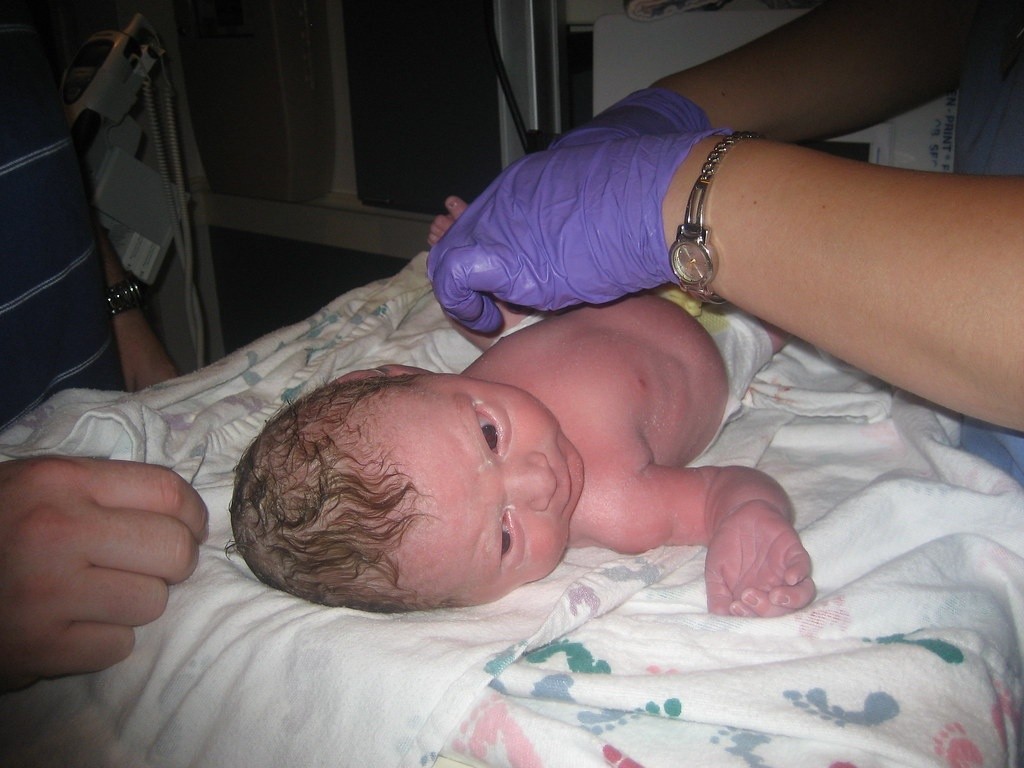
[424,128,734,336]
[545,88,712,134]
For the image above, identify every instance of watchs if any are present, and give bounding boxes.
[668,130,763,305]
[107,276,144,316]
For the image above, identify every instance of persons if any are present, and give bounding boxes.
[0,0,211,692]
[230,196,816,618]
[424,0,1024,485]
